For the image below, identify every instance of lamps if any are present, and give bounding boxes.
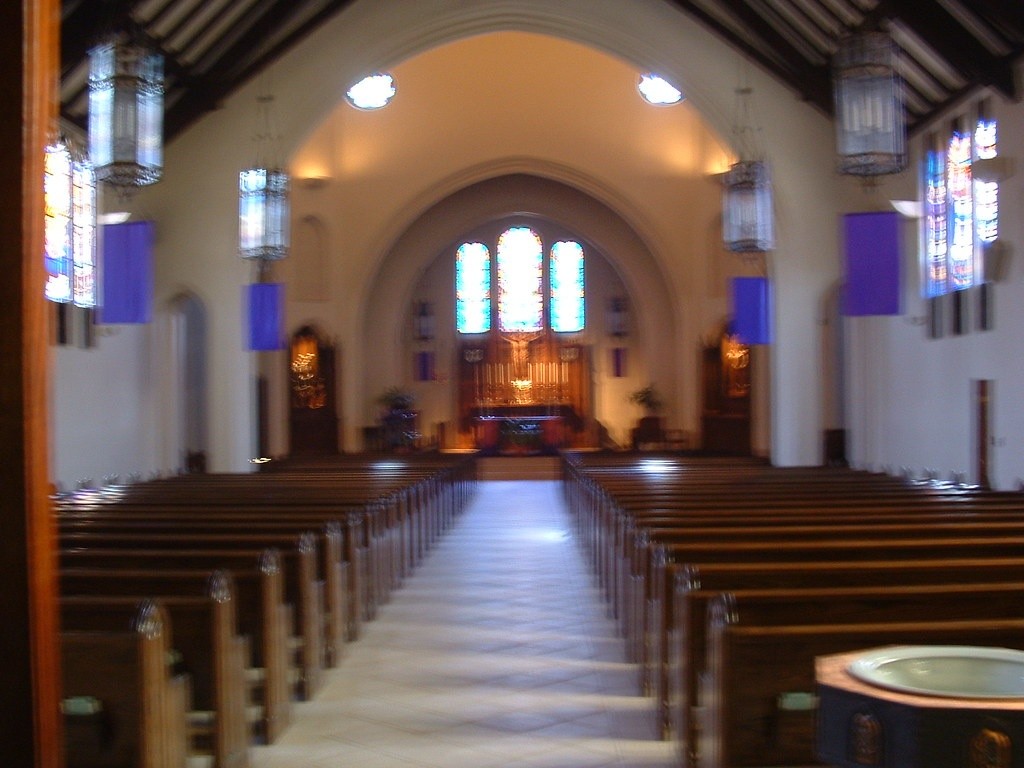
[236,66,289,264]
[84,1,166,187]
[720,69,777,253]
[830,0,908,182]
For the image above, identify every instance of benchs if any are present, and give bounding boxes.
[559,447,1024,768]
[48,446,483,768]
